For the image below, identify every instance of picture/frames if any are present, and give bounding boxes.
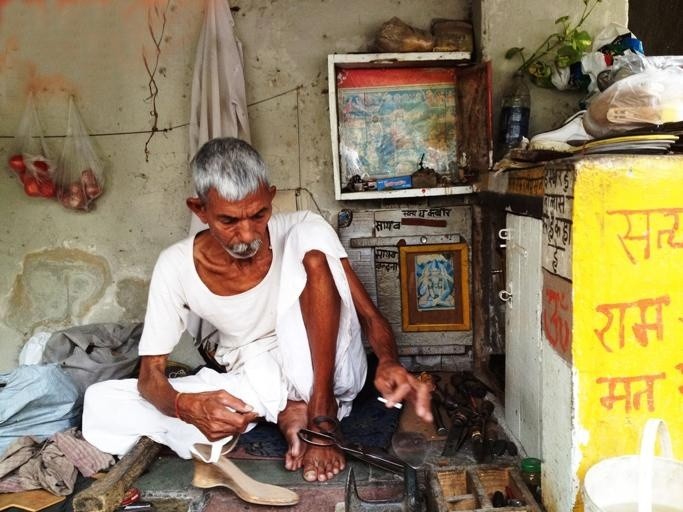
[398,242,473,334]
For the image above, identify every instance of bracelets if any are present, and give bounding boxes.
[173,390,183,420]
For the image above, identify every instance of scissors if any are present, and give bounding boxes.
[296,415,406,477]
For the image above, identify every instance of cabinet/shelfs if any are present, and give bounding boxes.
[482,152,683,512]
[323,50,472,205]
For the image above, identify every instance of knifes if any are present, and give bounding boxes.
[441,407,470,458]
[471,424,484,465]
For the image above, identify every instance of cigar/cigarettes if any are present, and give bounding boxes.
[377,397,403,411]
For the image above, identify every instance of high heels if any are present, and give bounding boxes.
[190,436,299,506]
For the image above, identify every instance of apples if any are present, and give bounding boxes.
[9,155,56,199]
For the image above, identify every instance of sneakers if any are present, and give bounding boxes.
[529,110,593,154]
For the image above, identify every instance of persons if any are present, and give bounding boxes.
[83,137,435,481]
[418,258,456,308]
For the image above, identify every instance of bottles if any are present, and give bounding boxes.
[522,457,543,504]
[494,71,531,160]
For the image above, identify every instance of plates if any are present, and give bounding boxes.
[568,133,680,153]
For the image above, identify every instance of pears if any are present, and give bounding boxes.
[56,170,102,209]
